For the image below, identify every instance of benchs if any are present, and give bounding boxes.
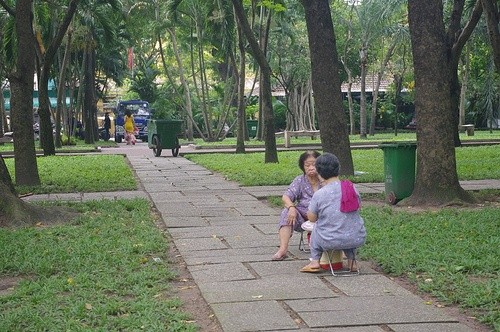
[283,130,322,150]
[458,123,474,136]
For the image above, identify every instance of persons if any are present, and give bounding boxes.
[105,112,111,141]
[272,150,326,260]
[124,109,136,145]
[300,152,367,273]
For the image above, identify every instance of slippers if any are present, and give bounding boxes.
[350,263,361,272]
[300,264,321,272]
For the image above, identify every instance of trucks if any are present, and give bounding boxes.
[111,100,155,143]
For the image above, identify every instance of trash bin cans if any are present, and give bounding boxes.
[245,119,258,139]
[145,118,185,157]
[378,140,417,205]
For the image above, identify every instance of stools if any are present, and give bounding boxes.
[297,229,311,253]
[326,249,360,277]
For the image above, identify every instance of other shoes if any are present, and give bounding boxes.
[272,250,289,261]
